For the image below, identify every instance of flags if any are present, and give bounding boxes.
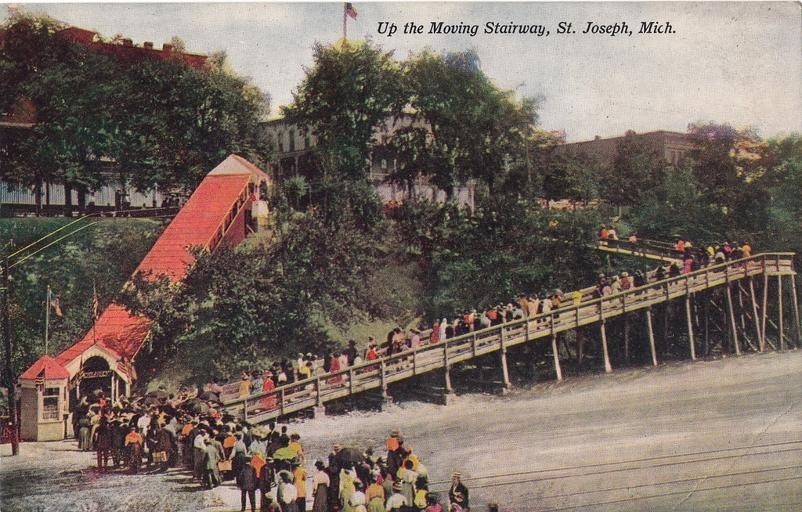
[346,3,358,21]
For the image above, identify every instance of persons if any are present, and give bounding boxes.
[63,405,501,512]
[70,183,753,406]
